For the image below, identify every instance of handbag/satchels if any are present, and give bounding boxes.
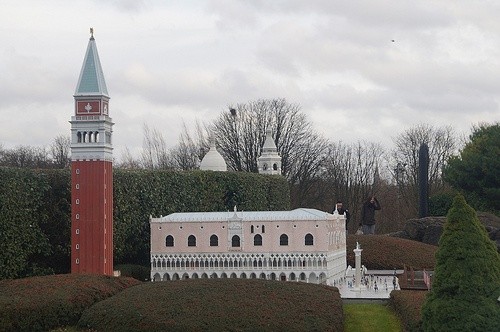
[356,226,363,234]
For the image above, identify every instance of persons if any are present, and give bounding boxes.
[359,193,382,235]
[332,202,350,233]
